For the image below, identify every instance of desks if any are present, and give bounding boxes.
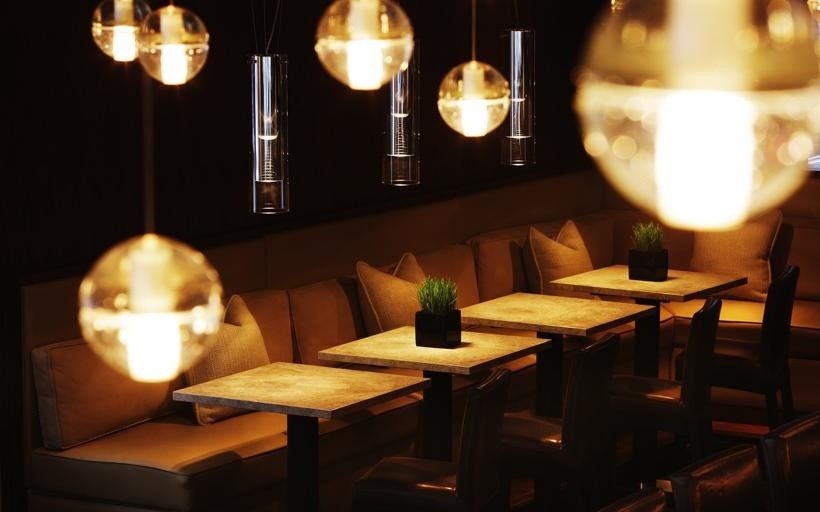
[550,264,748,380]
[459,291,656,420]
[171,361,431,507]
[317,325,552,459]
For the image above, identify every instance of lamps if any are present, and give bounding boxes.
[437,2,511,143]
[380,42,425,186]
[313,2,416,90]
[247,52,292,215]
[572,0,819,225]
[91,0,141,65]
[140,4,210,91]
[509,29,534,170]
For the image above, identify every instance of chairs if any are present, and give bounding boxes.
[641,298,723,503]
[351,378,505,510]
[675,268,803,442]
[502,330,619,507]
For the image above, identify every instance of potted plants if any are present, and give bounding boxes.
[413,279,462,346]
[626,223,669,281]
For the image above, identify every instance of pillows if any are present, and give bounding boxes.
[521,219,597,299]
[185,295,271,422]
[355,252,430,332]
[694,206,783,298]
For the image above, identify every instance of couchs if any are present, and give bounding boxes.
[2,175,816,508]
